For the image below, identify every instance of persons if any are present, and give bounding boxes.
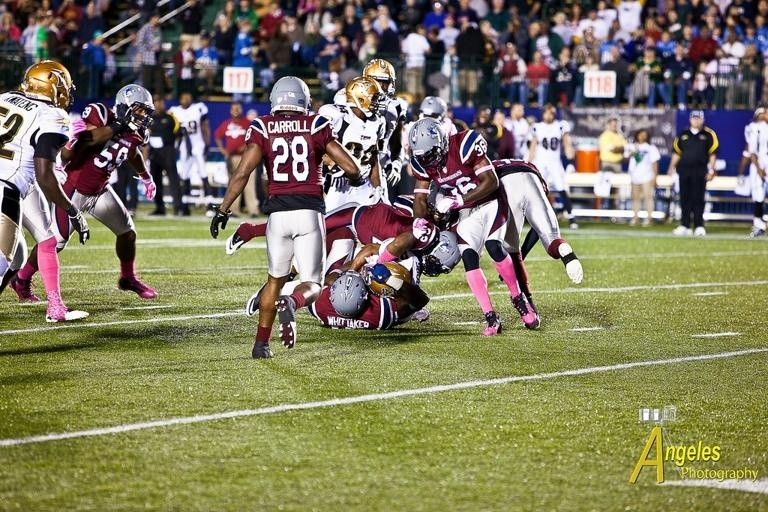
[591,119,628,227]
[0,60,90,289]
[117,163,136,218]
[12,120,90,322]
[490,157,584,321]
[446,103,471,132]
[8,82,158,302]
[214,100,263,219]
[210,74,360,360]
[166,91,215,218]
[748,113,768,236]
[332,60,406,188]
[245,76,396,318]
[503,104,532,160]
[529,103,579,230]
[308,244,429,330]
[668,110,721,237]
[737,108,765,181]
[408,118,541,337]
[223,202,463,278]
[624,130,661,229]
[145,93,185,218]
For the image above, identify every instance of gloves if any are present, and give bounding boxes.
[347,175,367,187]
[436,193,465,214]
[65,118,87,151]
[381,158,403,188]
[209,208,233,239]
[370,263,392,283]
[114,102,134,132]
[68,208,90,245]
[142,174,157,201]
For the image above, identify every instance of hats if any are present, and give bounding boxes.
[690,109,706,118]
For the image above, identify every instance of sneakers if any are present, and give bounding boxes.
[694,227,706,234]
[225,231,246,255]
[45,308,90,323]
[673,226,692,235]
[252,341,274,359]
[509,292,539,330]
[246,280,269,318]
[480,311,502,336]
[274,295,296,351]
[118,276,157,299]
[7,270,41,302]
[558,242,584,285]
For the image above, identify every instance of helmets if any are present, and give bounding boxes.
[363,58,396,88]
[114,84,156,132]
[419,95,448,116]
[408,117,449,167]
[329,269,369,318]
[23,59,76,115]
[269,76,312,116]
[345,76,389,118]
[420,230,462,277]
